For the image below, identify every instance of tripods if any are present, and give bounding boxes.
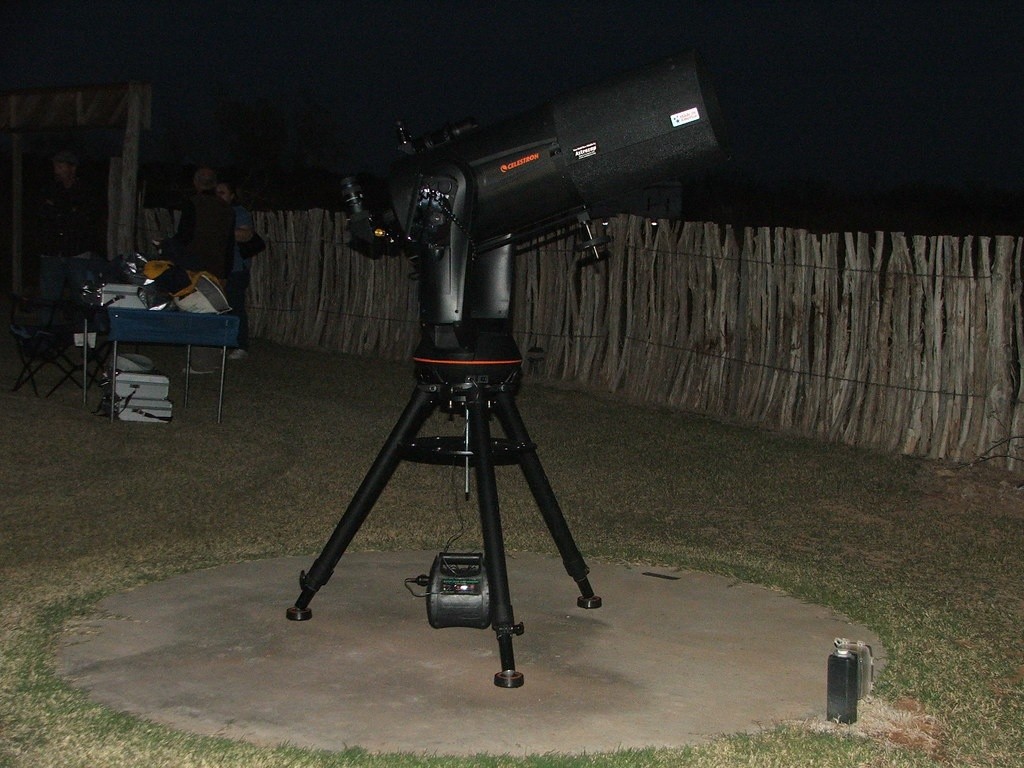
[287,333,603,688]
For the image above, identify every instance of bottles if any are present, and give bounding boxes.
[827,637,873,725]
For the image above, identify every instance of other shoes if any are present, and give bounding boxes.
[228,348,247,361]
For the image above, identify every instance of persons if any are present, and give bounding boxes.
[46,152,108,254]
[154,167,254,360]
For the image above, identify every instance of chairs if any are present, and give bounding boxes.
[7,254,139,399]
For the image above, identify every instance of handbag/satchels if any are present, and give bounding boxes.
[173,274,232,315]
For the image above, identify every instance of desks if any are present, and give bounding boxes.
[83,304,240,423]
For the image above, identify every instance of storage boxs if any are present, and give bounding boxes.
[115,373,169,400]
[102,282,146,309]
[118,400,172,422]
